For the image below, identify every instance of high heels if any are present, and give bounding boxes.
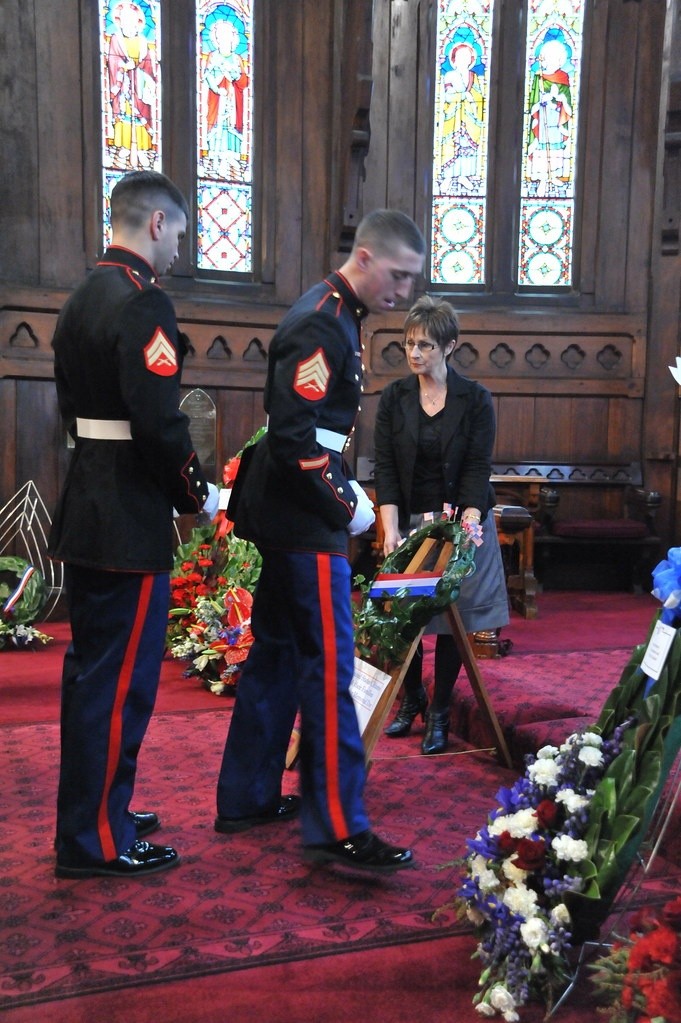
[421,704,451,754]
[384,686,428,738]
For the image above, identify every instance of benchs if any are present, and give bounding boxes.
[356,455,662,599]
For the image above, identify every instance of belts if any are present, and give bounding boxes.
[76,417,132,440]
[267,414,351,453]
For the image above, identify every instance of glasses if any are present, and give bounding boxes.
[402,340,443,353]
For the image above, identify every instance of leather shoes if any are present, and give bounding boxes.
[54,840,180,879]
[214,794,304,833]
[300,829,414,870]
[129,811,160,837]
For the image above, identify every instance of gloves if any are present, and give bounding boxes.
[196,482,219,526]
[348,480,375,509]
[346,496,376,537]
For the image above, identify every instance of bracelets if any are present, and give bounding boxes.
[464,515,481,523]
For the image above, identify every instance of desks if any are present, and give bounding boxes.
[370,475,549,621]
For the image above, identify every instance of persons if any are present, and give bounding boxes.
[213,209,427,874]
[43,170,219,880]
[372,295,509,756]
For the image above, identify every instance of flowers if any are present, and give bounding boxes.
[170,544,228,630]
[170,584,255,695]
[587,894,680,1023]
[1,626,52,651]
[456,718,642,1023]
